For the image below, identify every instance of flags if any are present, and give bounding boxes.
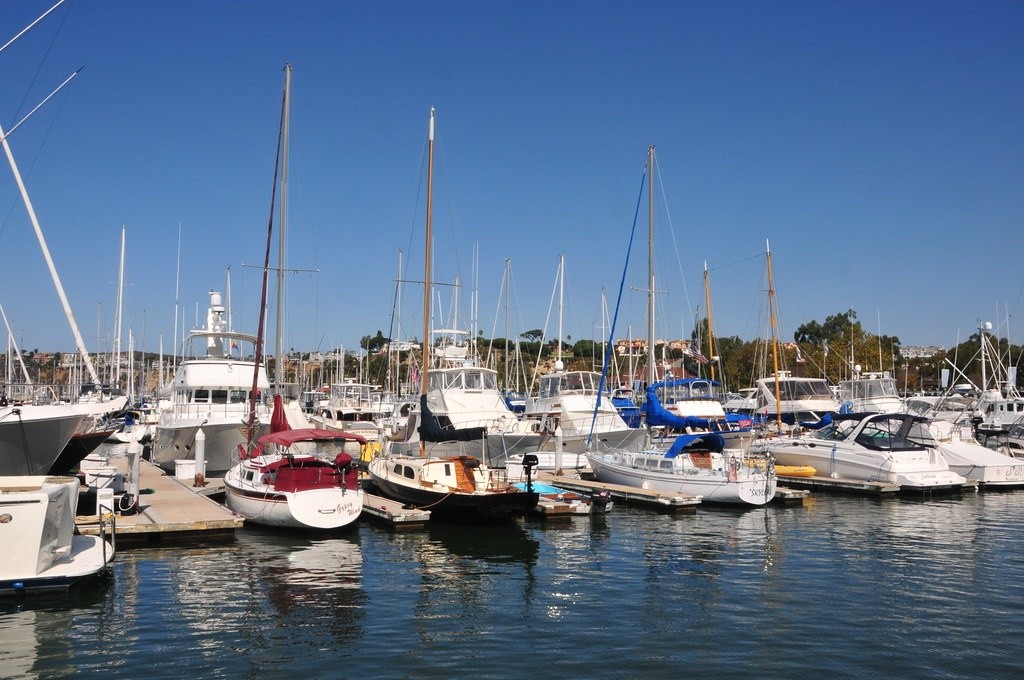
[231,338,239,352]
[687,330,709,365]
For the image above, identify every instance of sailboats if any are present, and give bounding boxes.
[831,298,1024,492]
[583,143,779,510]
[366,106,548,524]
[0,214,831,476]
[219,60,373,536]
[0,115,124,599]
